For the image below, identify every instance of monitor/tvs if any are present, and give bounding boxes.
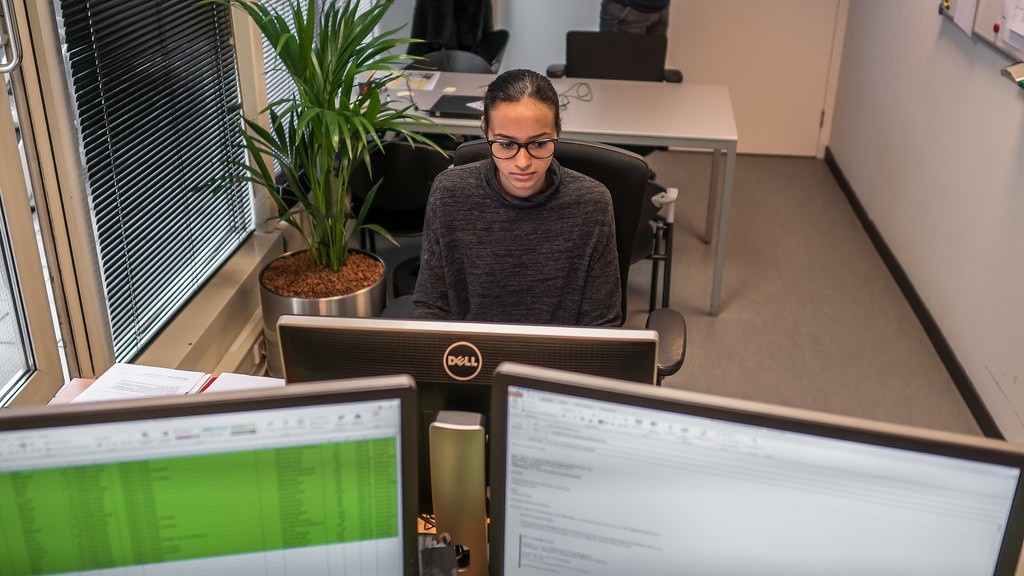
[0,313,1024,576]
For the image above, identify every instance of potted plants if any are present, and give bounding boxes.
[197,1,455,378]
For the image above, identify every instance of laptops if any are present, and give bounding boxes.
[428,94,484,120]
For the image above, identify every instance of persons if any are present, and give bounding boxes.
[411,69,625,329]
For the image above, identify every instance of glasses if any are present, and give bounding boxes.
[484,122,559,159]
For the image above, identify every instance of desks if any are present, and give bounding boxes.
[332,70,738,317]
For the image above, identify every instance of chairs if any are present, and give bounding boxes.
[546,0,685,154]
[630,166,678,314]
[453,138,650,328]
[399,0,510,74]
[345,137,454,257]
